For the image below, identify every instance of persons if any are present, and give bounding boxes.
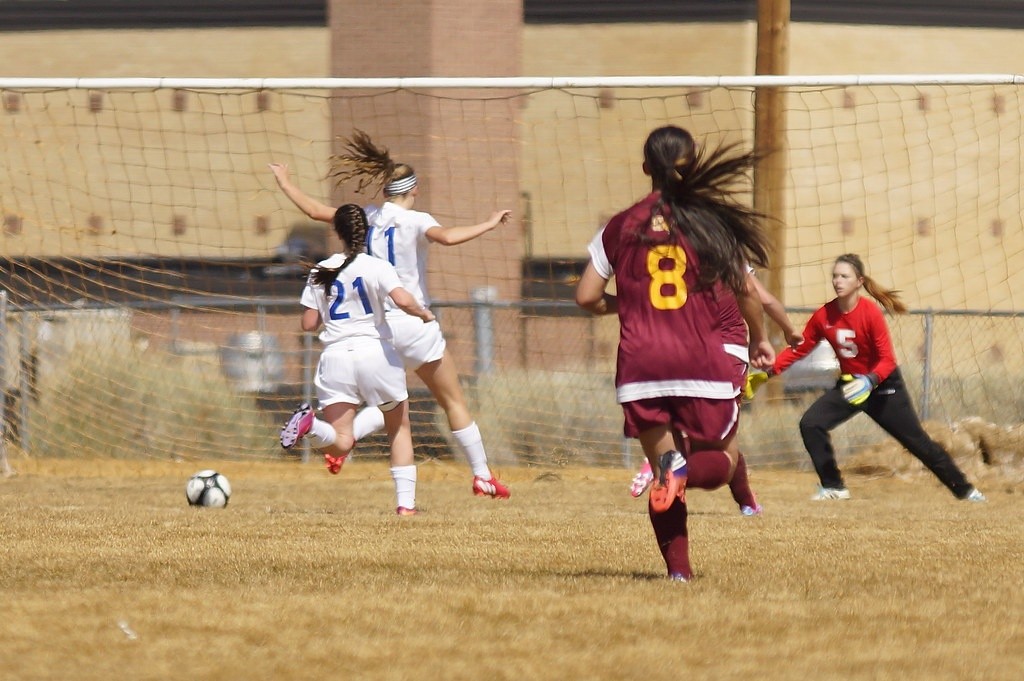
[281,203,435,516]
[268,127,513,499]
[631,266,805,515]
[576,126,783,583]
[743,253,985,501]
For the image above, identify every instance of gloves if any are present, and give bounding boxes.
[740,367,774,402]
[840,372,881,406]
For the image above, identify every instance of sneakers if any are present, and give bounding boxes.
[669,573,694,583]
[629,456,655,498]
[969,488,986,502]
[396,506,417,515]
[324,439,357,475]
[649,449,688,513]
[741,505,762,516]
[472,475,511,499]
[810,487,852,502]
[279,402,316,450]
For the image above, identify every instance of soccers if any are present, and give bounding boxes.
[184,468,232,510]
